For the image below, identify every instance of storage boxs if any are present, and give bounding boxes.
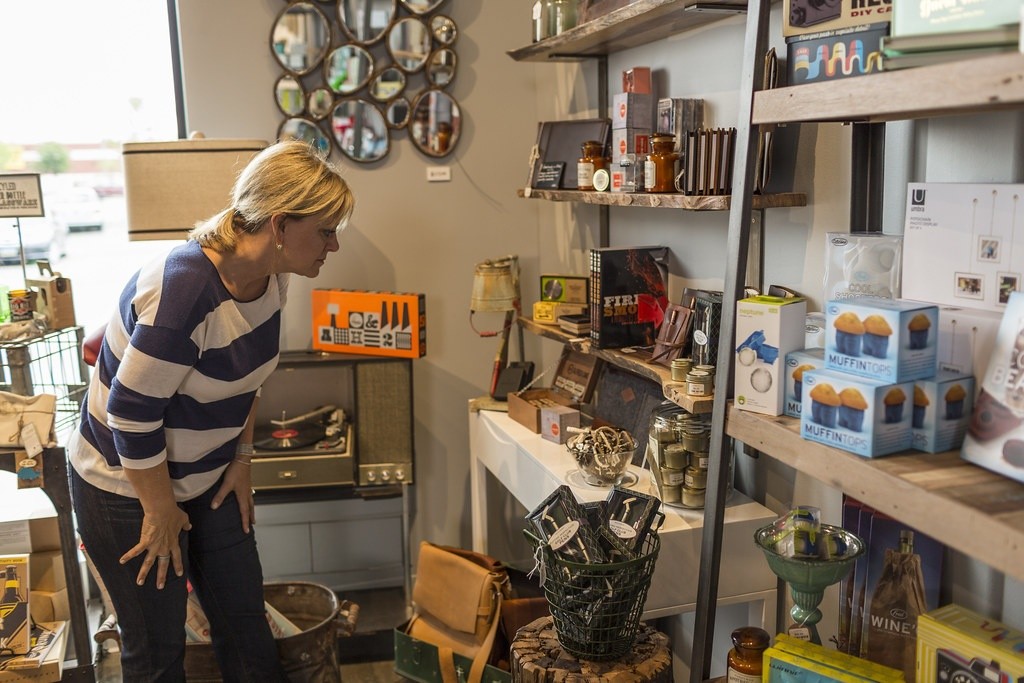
[762,633,905,683]
[311,289,428,359]
[506,390,581,444]
[916,603,1024,683]
[25,275,75,333]
[609,68,653,193]
[733,183,1024,483]
[0,517,90,683]
[782,0,1024,87]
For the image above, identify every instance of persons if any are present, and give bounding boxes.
[67,142,354,683]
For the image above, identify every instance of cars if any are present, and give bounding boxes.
[1,188,109,266]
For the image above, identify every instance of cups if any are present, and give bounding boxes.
[546,0,578,38]
[7,289,33,322]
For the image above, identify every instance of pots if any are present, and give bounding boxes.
[183,581,360,683]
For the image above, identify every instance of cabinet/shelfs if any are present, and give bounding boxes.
[506,0,1024,683]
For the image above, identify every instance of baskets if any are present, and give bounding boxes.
[525,501,660,664]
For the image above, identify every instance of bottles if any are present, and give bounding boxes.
[725,625,771,683]
[644,132,677,194]
[577,140,605,191]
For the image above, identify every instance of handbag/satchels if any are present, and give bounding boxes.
[406,540,518,683]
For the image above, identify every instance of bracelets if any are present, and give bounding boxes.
[235,444,254,465]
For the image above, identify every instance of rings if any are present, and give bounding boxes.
[158,555,170,559]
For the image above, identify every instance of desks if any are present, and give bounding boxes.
[468,400,779,648]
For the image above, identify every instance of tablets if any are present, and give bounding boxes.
[531,118,612,189]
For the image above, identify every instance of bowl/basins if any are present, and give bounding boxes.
[565,434,640,488]
